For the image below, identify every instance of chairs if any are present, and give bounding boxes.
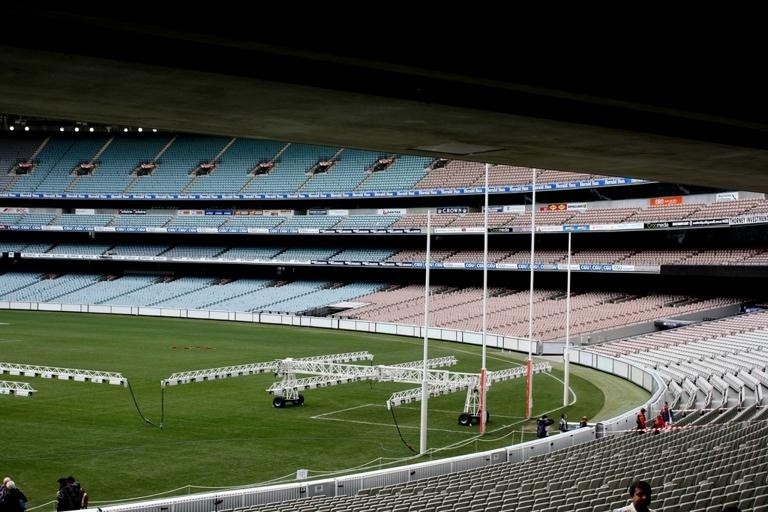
[216,423,768,512]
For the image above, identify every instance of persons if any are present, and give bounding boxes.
[558,413,569,434]
[0,477,11,503]
[635,407,647,434]
[3,481,27,512]
[658,401,673,424]
[651,415,665,429]
[610,481,657,512]
[56,477,80,512]
[66,476,82,510]
[535,415,555,438]
[578,416,588,428]
